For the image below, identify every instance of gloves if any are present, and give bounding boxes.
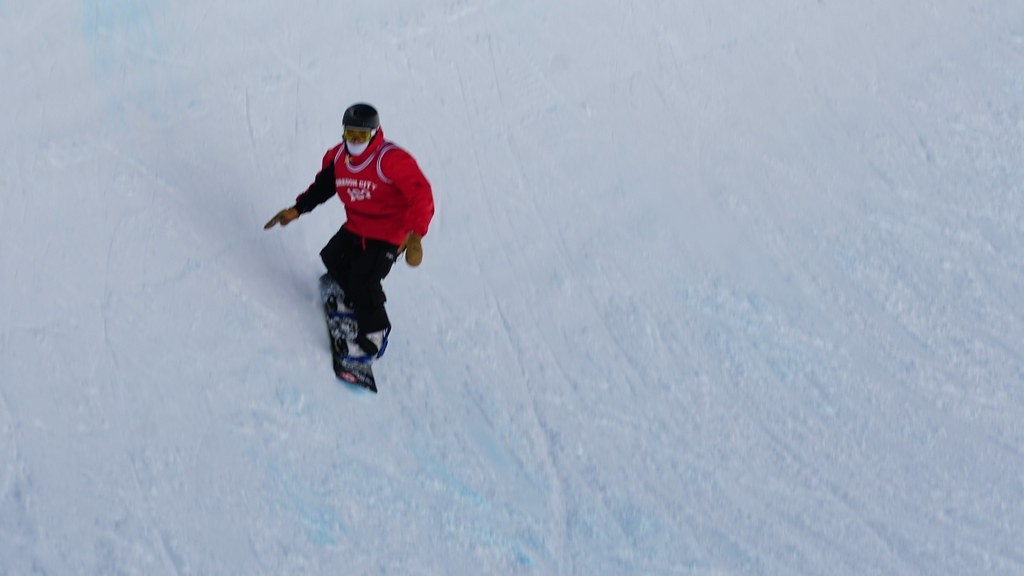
[397,231,423,265]
[264,207,299,229]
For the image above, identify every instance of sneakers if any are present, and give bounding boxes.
[328,294,357,319]
[338,324,391,370]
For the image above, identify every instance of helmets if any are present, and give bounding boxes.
[342,104,380,129]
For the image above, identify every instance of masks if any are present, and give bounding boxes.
[346,130,377,156]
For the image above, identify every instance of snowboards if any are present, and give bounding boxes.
[320,270,378,394]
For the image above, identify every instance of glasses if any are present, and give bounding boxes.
[344,130,371,143]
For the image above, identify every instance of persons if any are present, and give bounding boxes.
[264,103,434,367]
